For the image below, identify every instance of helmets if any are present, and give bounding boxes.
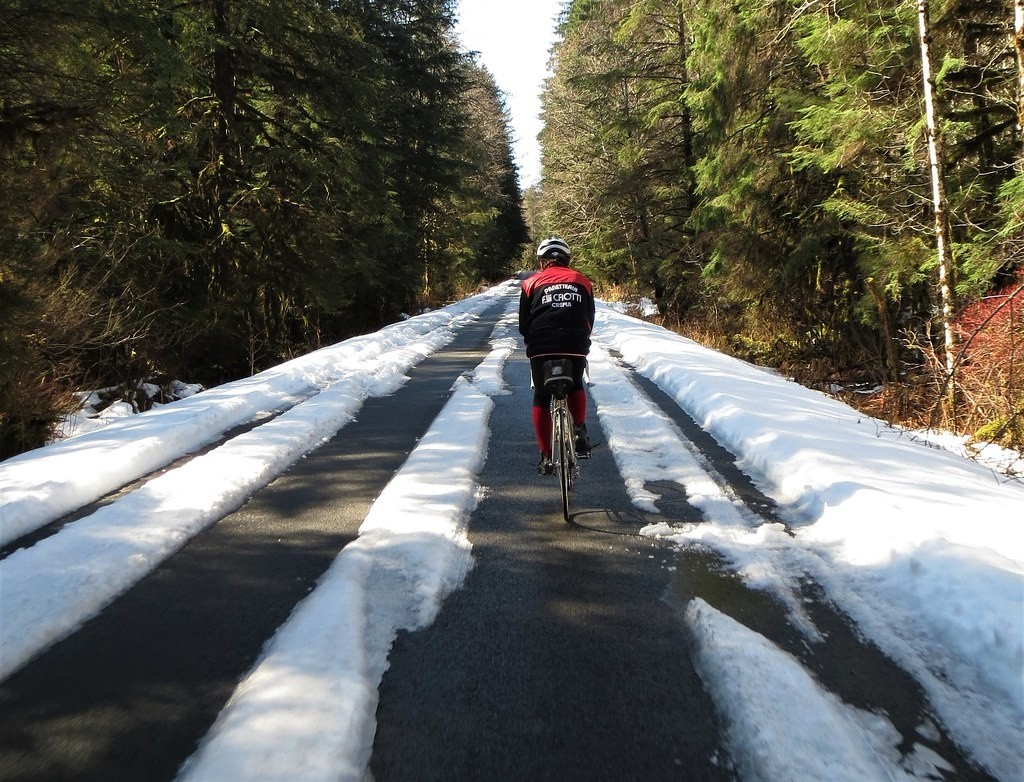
[537,237,572,262]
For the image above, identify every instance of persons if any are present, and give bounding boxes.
[519,237,595,476]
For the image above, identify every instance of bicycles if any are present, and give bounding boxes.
[528,361,595,521]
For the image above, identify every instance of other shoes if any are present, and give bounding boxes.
[538,450,553,475]
[573,422,592,452]
